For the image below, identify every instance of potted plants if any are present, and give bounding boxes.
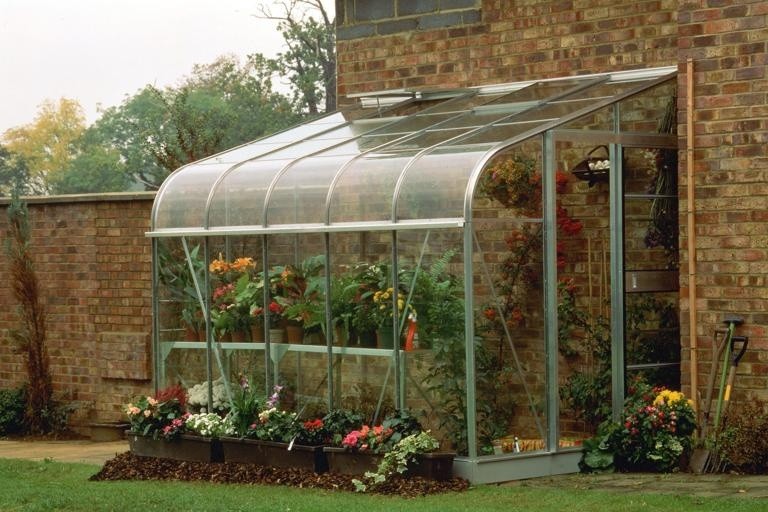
[155,240,418,350]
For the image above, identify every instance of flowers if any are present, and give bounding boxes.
[604,375,696,470]
[477,156,538,203]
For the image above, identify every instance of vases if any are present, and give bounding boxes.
[495,189,528,207]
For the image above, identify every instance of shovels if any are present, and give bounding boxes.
[689,328,731,475]
[711,336,748,473]
